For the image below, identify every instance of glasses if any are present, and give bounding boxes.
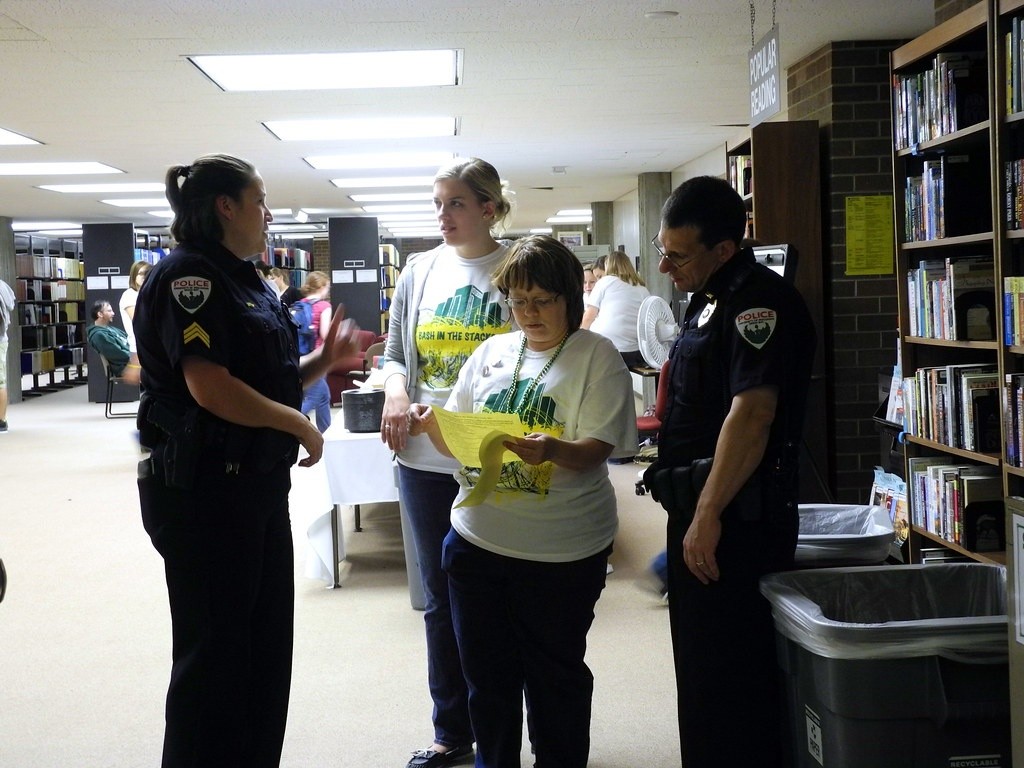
[504,291,563,309]
[651,234,721,269]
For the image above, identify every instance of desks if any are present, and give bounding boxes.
[306,371,429,589]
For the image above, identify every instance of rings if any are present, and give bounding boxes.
[385,425,390,428]
[696,562,704,565]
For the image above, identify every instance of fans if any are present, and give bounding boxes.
[637,295,685,369]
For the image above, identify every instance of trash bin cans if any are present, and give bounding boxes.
[392,464,426,610]
[759,561,1012,768]
[794,503,894,568]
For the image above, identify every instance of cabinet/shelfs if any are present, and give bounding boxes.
[329,217,409,339]
[725,120,828,503]
[889,0,1024,572]
[81,223,175,405]
[1,216,88,406]
[255,235,314,292]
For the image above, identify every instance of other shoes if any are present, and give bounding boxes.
[405,743,474,768]
[0,419,8,433]
[636,568,670,605]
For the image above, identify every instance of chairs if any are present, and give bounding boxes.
[100,355,154,419]
[635,360,670,461]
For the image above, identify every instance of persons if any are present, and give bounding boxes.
[86,299,141,377]
[642,176,814,768]
[132,154,361,768]
[576,248,650,367]
[0,279,14,431]
[119,261,157,362]
[381,157,519,768]
[407,235,637,768]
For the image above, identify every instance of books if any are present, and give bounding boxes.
[1004,15,1024,115]
[1004,276,1024,346]
[20,347,84,374]
[1004,158,1024,229]
[1003,374,1024,468]
[18,280,85,301]
[892,50,962,151]
[373,244,400,334]
[133,246,170,266]
[908,456,1006,552]
[903,255,990,341]
[21,325,82,349]
[16,255,85,278]
[903,154,985,241]
[18,302,78,325]
[903,361,999,453]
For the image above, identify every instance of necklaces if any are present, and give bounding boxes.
[507,334,569,414]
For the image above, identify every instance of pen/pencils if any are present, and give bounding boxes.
[281,300,292,319]
[682,320,689,337]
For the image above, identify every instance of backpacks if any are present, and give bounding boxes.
[288,298,323,356]
